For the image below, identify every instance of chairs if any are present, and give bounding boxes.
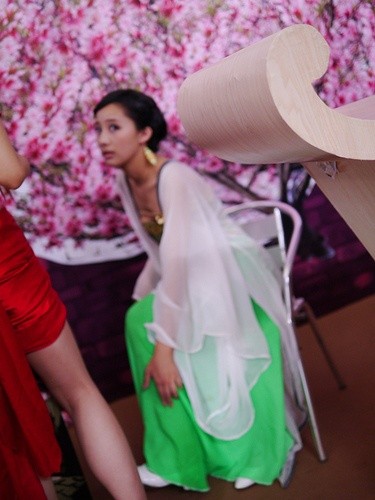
[223,199,345,464]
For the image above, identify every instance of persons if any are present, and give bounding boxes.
[92,88,310,493]
[0,119,148,499]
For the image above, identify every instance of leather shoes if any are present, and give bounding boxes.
[137,463,172,488]
[234,476,255,490]
